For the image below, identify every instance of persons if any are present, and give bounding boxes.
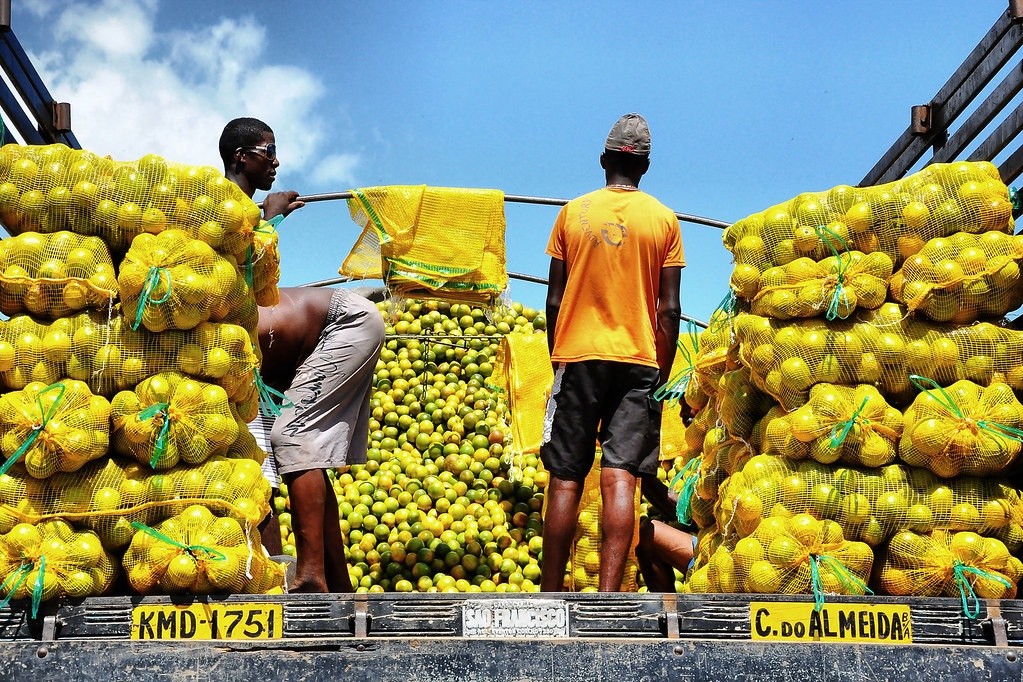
[238,281,386,593]
[216,117,305,230]
[539,111,685,589]
[637,375,712,592]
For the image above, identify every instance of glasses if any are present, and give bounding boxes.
[241,143,276,161]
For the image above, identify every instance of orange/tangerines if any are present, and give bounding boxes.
[0,140,1022,604]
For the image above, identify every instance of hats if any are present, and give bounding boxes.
[604,112,651,156]
